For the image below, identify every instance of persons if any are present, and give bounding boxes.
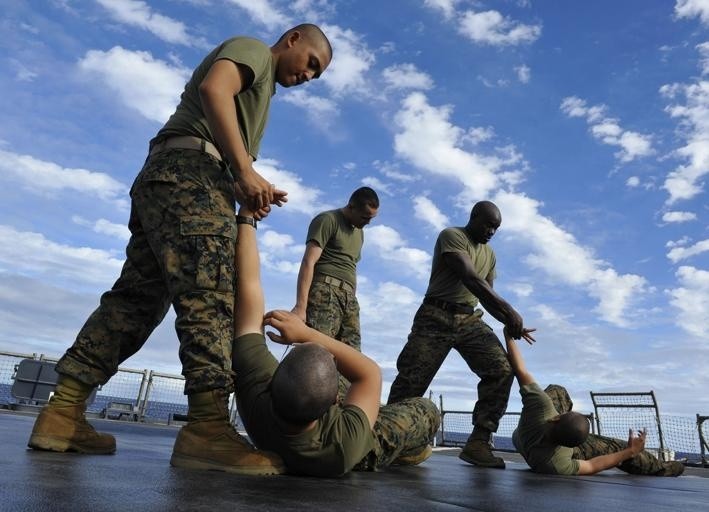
[289,187,381,409]
[502,325,687,479]
[386,200,524,470]
[26,21,335,478]
[232,187,442,479]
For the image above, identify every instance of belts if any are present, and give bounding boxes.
[423,297,473,314]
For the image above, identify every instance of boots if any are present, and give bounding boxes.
[170,385,285,476]
[28,373,116,454]
[459,426,505,468]
[391,444,432,465]
[653,461,684,476]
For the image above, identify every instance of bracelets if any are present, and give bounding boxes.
[235,213,259,230]
